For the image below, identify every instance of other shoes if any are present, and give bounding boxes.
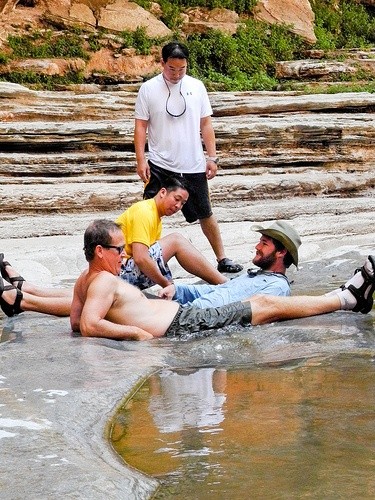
[217,258,243,273]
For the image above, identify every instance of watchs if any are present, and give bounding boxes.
[208,157,220,163]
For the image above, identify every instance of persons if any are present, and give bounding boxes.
[133,42,243,273]
[0,220,301,317]
[70,219,375,341]
[113,178,234,291]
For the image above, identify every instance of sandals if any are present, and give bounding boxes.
[340,255,375,314]
[0,253,25,291]
[0,276,24,317]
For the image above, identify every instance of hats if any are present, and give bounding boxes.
[250,220,302,271]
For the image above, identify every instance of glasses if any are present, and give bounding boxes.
[100,244,125,255]
